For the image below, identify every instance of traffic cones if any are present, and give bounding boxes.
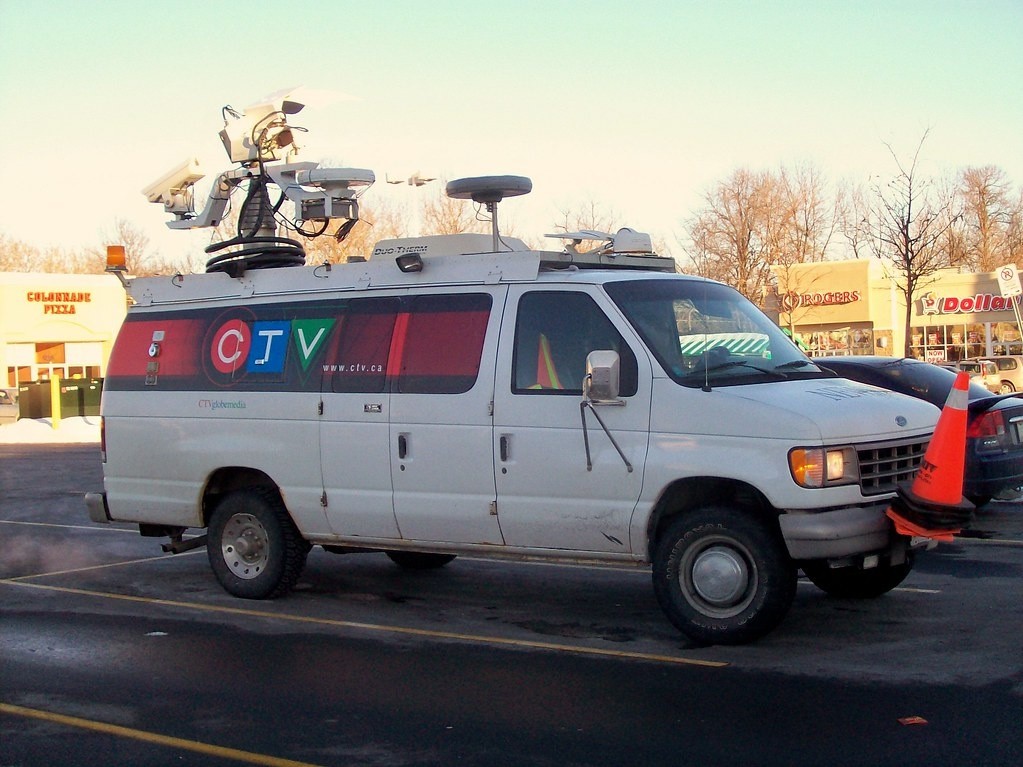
[884,367,976,544]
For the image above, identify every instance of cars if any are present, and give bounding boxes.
[815,354,1023,509]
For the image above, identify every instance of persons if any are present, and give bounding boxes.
[641,315,689,374]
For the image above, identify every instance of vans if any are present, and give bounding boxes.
[87,98,946,649]
